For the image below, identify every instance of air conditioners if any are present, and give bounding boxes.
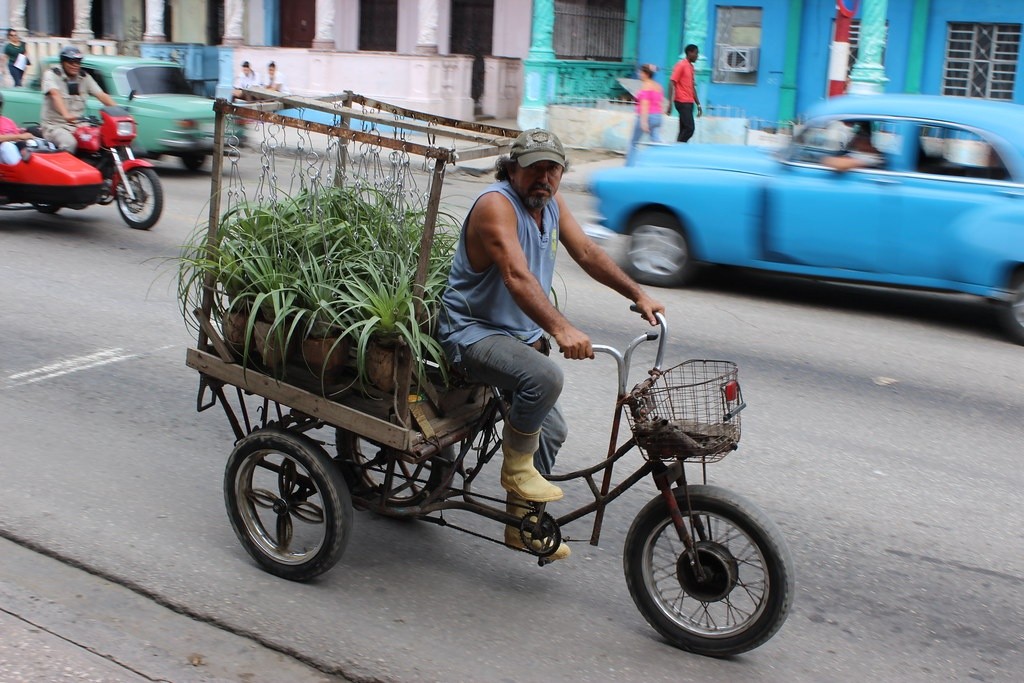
[717,44,759,75]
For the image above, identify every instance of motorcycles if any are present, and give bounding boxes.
[1,90,160,228]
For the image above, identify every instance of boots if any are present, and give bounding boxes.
[503,491,571,561]
[500,410,565,504]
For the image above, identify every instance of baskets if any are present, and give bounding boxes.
[617,359,743,465]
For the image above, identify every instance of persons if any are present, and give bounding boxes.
[813,116,886,173]
[260,60,292,94]
[231,60,259,104]
[437,127,665,564]
[666,44,703,142]
[0,93,34,165]
[0,28,32,86]
[623,63,664,165]
[41,46,128,154]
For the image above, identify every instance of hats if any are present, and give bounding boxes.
[508,127,568,169]
[60,46,85,59]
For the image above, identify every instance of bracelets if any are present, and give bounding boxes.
[698,105,701,107]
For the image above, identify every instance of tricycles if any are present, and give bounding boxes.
[184,90,793,662]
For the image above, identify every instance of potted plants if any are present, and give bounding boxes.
[155,173,468,397]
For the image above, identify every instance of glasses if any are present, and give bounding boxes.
[9,34,17,37]
[64,58,81,64]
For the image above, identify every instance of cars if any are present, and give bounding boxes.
[580,94,1023,339]
[31,55,243,174]
